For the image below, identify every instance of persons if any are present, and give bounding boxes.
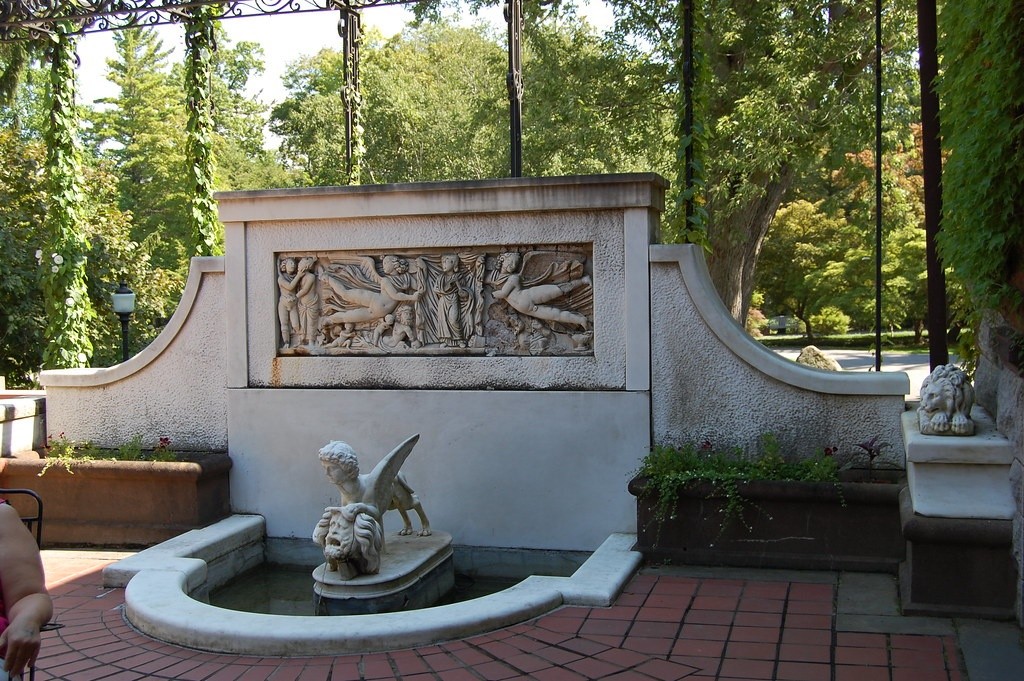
[287,257,320,347]
[317,440,360,485]
[0,496,54,681]
[475,251,593,328]
[278,260,307,349]
[313,501,382,580]
[434,254,467,348]
[316,255,428,351]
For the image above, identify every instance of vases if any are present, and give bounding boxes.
[3,448,233,547]
[627,467,907,574]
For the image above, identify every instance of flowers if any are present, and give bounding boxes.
[623,437,846,552]
[38,431,185,478]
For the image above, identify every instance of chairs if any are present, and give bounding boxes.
[0,488,66,681]
